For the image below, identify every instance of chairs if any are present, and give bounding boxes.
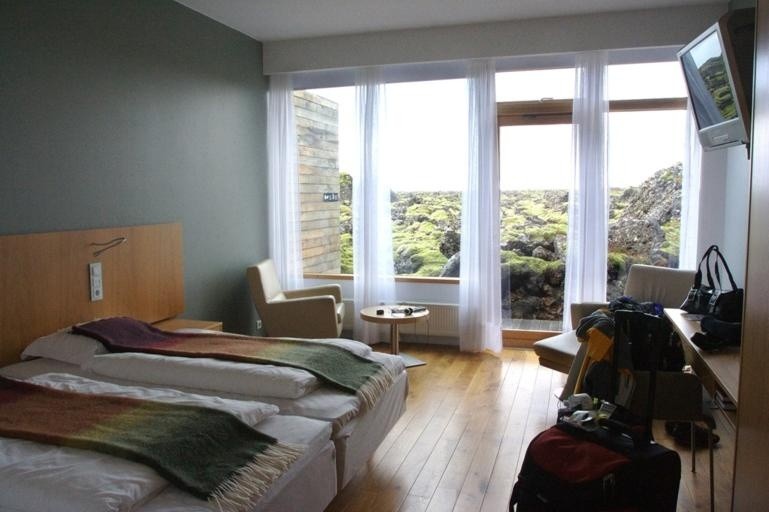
[534,264,698,376]
[616,326,716,512]
[245,257,346,339]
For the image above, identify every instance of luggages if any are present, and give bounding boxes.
[507,298,682,512]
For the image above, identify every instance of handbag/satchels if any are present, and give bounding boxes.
[679,245,740,321]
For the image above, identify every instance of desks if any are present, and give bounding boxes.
[661,307,743,432]
[360,304,431,368]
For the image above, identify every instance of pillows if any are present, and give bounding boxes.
[20,316,119,365]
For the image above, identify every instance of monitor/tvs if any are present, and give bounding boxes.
[677,7,755,151]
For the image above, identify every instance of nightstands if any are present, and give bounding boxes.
[151,319,224,331]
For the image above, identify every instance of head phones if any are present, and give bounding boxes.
[392,307,413,315]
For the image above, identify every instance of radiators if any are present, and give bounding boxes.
[403,302,460,339]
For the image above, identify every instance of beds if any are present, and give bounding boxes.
[0,361,338,511]
[21,326,407,490]
